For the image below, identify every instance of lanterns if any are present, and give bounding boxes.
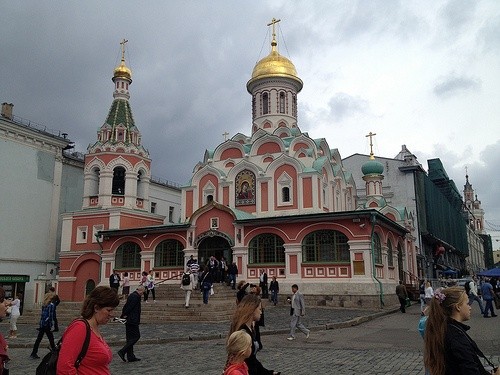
[438,247,446,256]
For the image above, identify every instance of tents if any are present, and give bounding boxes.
[476,267,500,277]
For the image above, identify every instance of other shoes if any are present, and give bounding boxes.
[306,330,311,339]
[30,353,41,358]
[36,328,40,331]
[52,329,59,332]
[9,336,17,339]
[287,336,296,341]
[117,350,127,362]
[127,358,141,362]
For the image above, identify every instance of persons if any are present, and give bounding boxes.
[139,269,158,304]
[117,285,145,363]
[222,329,252,375]
[259,269,270,299]
[466,276,500,319]
[180,270,195,308]
[0,294,22,339]
[269,275,279,306]
[395,280,412,313]
[228,294,281,374]
[109,268,121,295]
[0,335,10,375]
[236,279,265,351]
[186,254,200,290]
[55,286,120,375]
[418,285,500,375]
[36,287,61,333]
[199,266,213,304]
[286,284,310,341]
[120,272,131,300]
[206,255,239,289]
[419,281,435,317]
[29,293,58,358]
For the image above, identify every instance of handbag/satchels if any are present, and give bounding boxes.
[36,318,91,375]
[6,306,13,313]
[210,287,214,296]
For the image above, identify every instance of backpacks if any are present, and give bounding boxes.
[181,272,192,286]
[464,280,475,294]
